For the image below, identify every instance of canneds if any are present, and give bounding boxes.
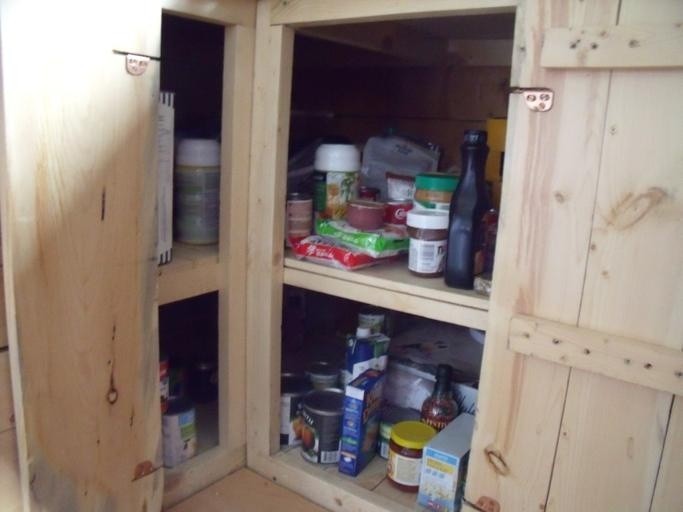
[384,198,414,226]
[387,420,438,494]
[414,174,459,204]
[407,211,448,278]
[304,357,341,389]
[300,386,346,465]
[358,186,382,203]
[161,396,198,468]
[160,340,171,415]
[279,372,309,448]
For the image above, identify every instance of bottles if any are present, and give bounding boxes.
[444,131,489,289]
[406,175,459,276]
[175,138,219,244]
[421,364,458,431]
[158,361,197,466]
[313,144,359,222]
[278,361,437,492]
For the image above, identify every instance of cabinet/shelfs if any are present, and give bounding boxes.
[160,0,249,507]
[243,1,520,512]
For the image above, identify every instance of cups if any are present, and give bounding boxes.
[346,200,386,231]
